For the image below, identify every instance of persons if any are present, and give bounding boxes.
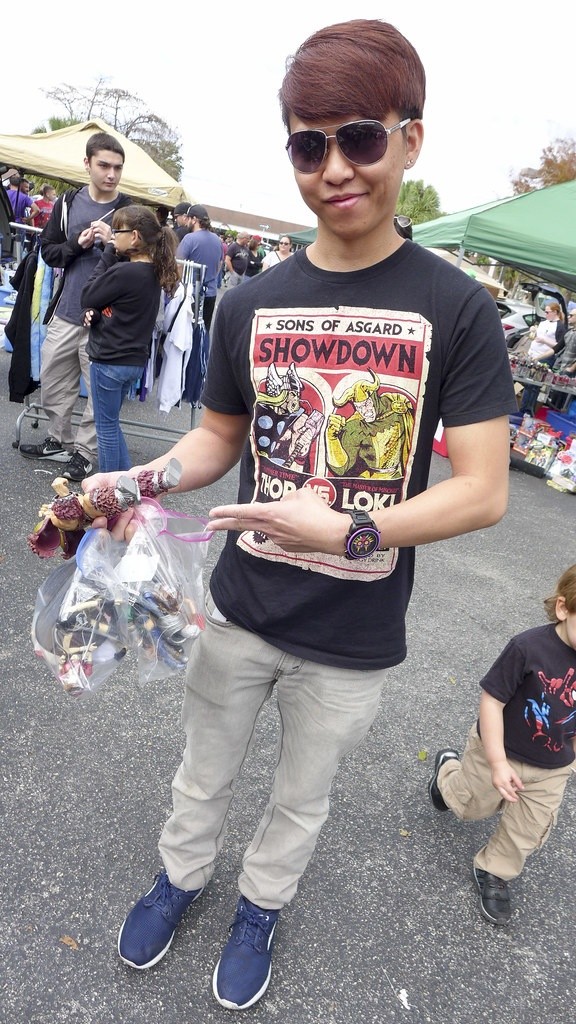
[519,302,567,418]
[176,204,225,330]
[6,176,40,246]
[173,202,192,242]
[80,205,180,473]
[217,232,234,288]
[19,179,34,237]
[534,308,576,410]
[19,133,133,481]
[30,186,56,228]
[27,457,182,560]
[81,19,518,1009]
[56,583,196,695]
[227,231,264,288]
[427,564,576,925]
[261,236,293,271]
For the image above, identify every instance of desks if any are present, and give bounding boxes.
[511,372,576,418]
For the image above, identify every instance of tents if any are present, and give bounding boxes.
[0,116,198,211]
[411,179,576,294]
[278,227,318,246]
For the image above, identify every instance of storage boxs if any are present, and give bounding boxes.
[545,410,576,442]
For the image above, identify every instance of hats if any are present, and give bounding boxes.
[185,204,210,221]
[174,202,192,217]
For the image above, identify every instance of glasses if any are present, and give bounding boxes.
[279,242,290,245]
[174,214,181,219]
[569,314,574,318]
[285,118,411,174]
[545,311,549,314]
[112,228,142,241]
[395,214,412,228]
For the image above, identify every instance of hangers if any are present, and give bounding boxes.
[32,227,39,252]
[173,256,206,287]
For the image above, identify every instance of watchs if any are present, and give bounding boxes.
[343,509,380,560]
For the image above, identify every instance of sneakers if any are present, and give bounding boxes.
[117,869,204,970]
[473,868,512,925]
[19,437,74,463]
[61,450,93,481]
[428,748,459,812]
[213,894,281,1010]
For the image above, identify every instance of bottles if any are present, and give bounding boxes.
[529,324,537,339]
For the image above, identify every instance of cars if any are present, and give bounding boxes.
[495,297,547,348]
